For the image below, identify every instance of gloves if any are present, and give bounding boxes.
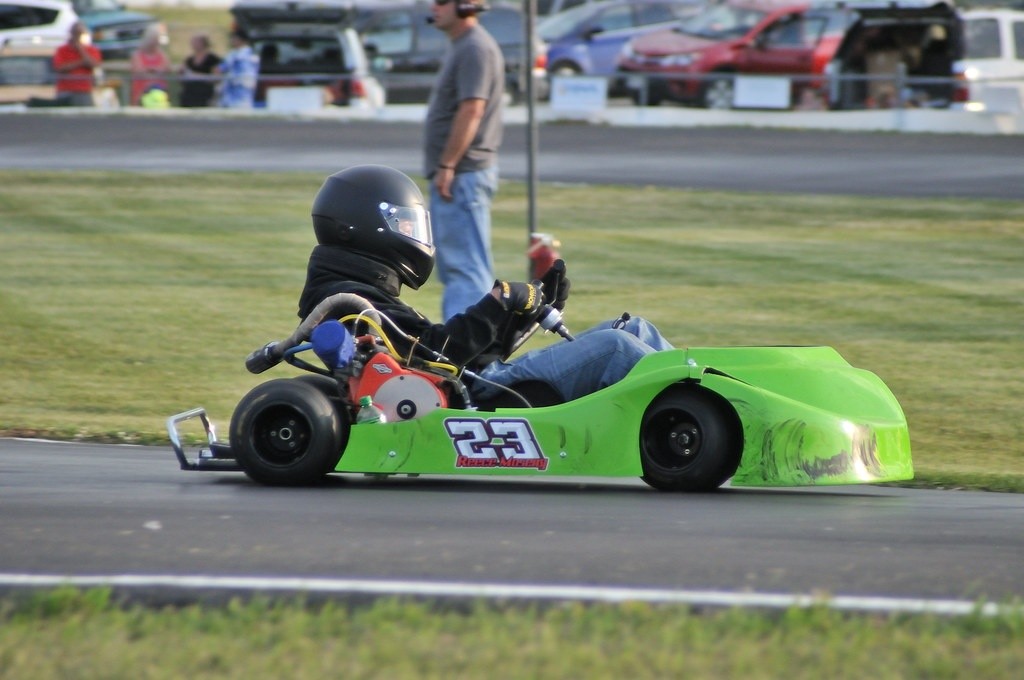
[539,266,571,311]
[492,278,547,323]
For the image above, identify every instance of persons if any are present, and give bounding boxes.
[177,34,224,107]
[211,28,261,108]
[129,22,180,106]
[909,24,951,107]
[424,0,506,323]
[298,164,674,413]
[54,23,103,107]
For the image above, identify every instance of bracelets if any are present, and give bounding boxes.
[437,163,455,170]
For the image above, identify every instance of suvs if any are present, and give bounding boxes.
[0,0,155,109]
[234,0,1024,113]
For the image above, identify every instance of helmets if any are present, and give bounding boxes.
[312,164,436,291]
